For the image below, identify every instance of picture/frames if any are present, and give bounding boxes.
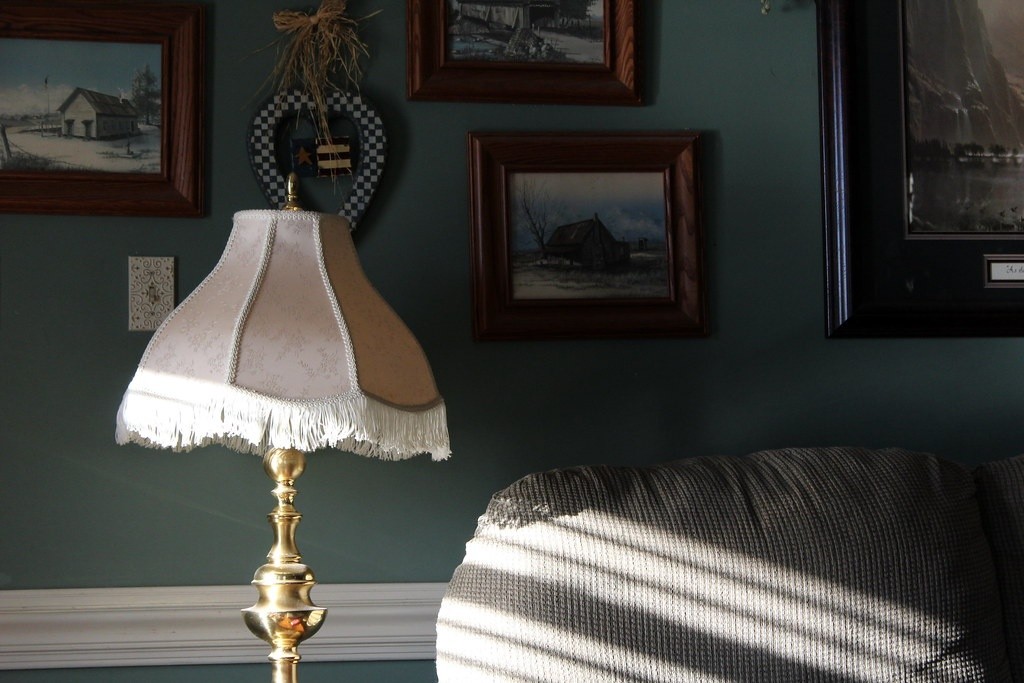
[815,1,1024,341]
[0,0,214,221]
[406,0,646,106]
[467,129,716,340]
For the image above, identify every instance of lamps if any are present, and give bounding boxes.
[114,172,451,683]
